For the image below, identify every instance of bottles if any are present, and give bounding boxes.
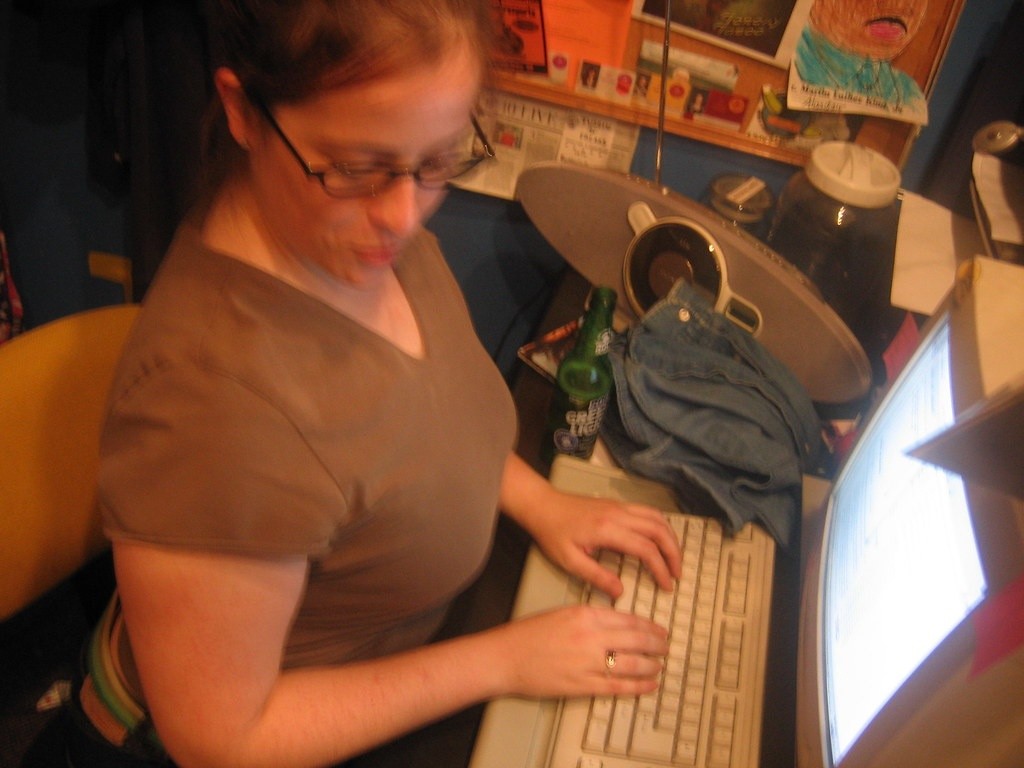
[693,170,779,241]
[538,285,618,465]
[766,140,904,338]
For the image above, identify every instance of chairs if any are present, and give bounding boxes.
[0,306,145,768]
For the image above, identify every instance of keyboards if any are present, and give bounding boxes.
[539,513,776,768]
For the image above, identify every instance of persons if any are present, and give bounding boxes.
[66,1,682,768]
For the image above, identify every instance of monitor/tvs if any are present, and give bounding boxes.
[793,254,1024,768]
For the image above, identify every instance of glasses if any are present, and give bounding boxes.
[252,95,496,200]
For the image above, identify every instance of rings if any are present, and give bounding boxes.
[605,650,617,677]
